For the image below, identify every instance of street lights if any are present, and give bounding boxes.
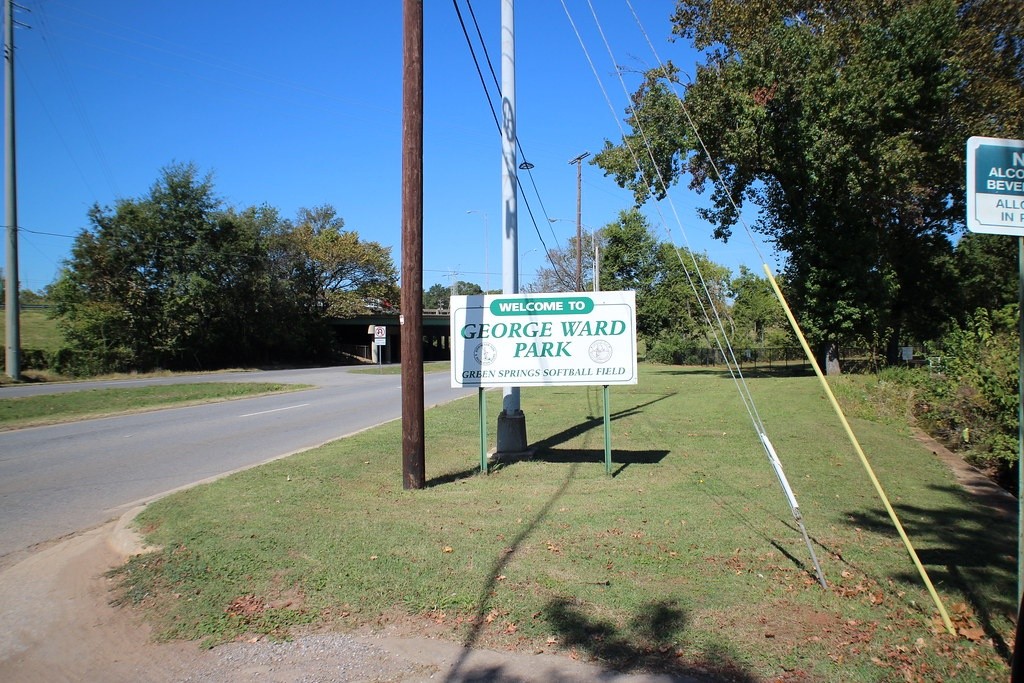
[548,218,596,292]
[466,209,491,295]
[520,248,538,292]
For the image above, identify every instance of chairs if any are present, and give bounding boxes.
[926,357,947,376]
